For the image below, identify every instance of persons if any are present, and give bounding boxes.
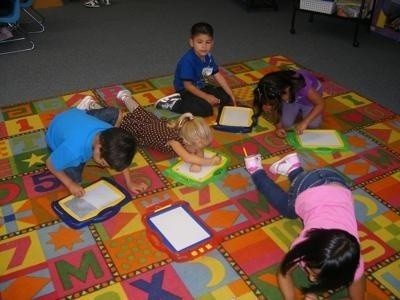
[155,21,238,117]
[43,95,149,200]
[244,149,366,299]
[74,89,222,173]
[256,69,326,138]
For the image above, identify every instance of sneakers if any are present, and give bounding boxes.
[154,93,182,111]
[0,29,12,42]
[244,154,262,175]
[269,152,302,178]
[76,96,94,111]
[115,89,132,100]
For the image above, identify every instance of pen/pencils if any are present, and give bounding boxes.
[155,204,172,213]
[243,147,248,157]
